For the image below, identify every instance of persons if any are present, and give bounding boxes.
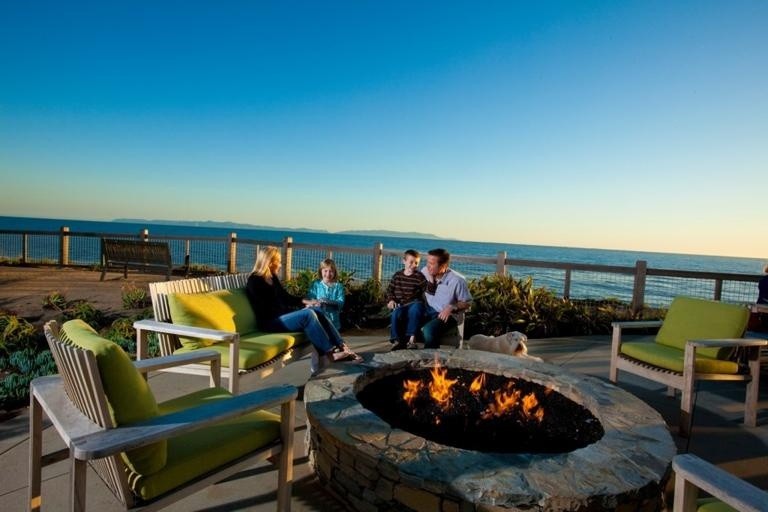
[420,248,473,350]
[306,257,346,334]
[756,264,767,319]
[244,245,362,362]
[384,248,433,350]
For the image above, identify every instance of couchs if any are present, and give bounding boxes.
[131,273,328,395]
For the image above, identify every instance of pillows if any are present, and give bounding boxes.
[653,295,751,360]
[167,287,258,350]
[57,317,168,475]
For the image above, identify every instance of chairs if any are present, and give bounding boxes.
[671,452,768,512]
[27,347,298,512]
[608,319,768,439]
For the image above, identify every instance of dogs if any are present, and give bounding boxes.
[467,331,544,364]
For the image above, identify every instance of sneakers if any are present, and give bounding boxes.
[326,343,365,364]
[390,339,418,351]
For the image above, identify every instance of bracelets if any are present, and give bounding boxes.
[453,304,459,311]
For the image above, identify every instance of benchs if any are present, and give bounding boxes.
[96,237,174,281]
